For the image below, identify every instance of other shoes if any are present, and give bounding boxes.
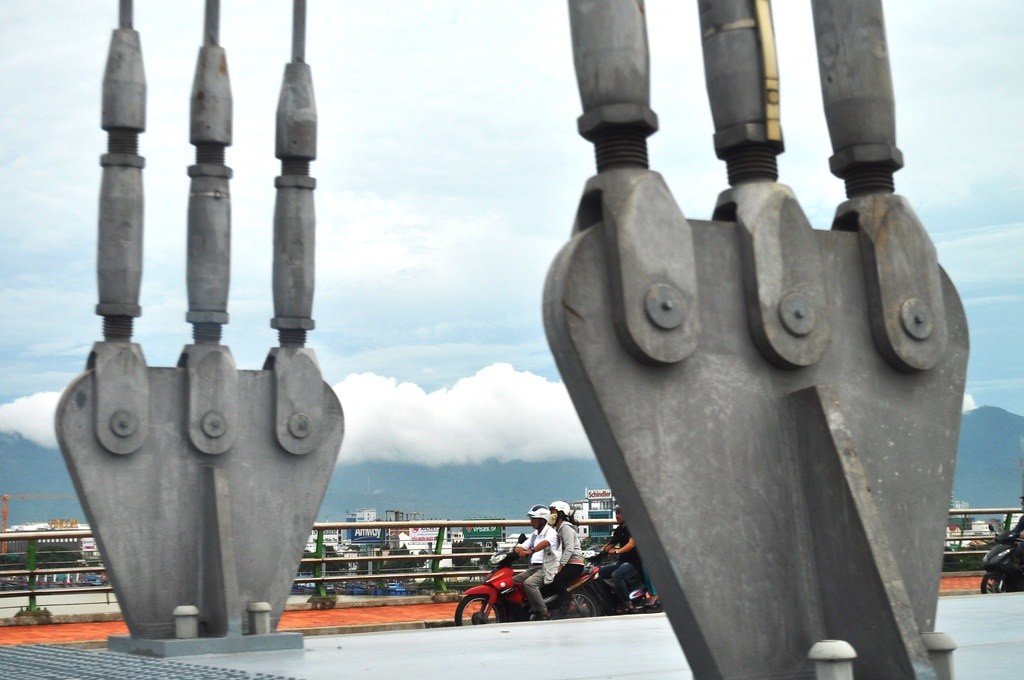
[536,612,551,620]
[616,604,633,614]
[565,600,577,613]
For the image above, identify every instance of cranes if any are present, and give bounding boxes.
[0,494,80,554]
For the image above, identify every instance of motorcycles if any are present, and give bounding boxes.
[979,521,1024,593]
[454,533,608,626]
[573,544,664,618]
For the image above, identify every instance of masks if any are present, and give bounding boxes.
[547,513,557,525]
[1021,506,1024,512]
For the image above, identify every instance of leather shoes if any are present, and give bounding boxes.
[645,595,659,606]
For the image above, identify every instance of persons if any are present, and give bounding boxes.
[1012,495,1024,540]
[600,502,659,613]
[508,497,585,621]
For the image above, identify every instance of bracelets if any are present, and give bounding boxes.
[531,548,535,553]
[615,550,618,554]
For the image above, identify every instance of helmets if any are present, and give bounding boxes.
[526,504,550,522]
[612,504,621,512]
[549,500,570,516]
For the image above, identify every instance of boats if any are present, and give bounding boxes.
[0,575,110,590]
[290,581,413,594]
